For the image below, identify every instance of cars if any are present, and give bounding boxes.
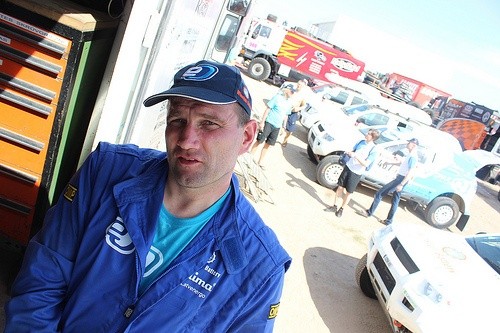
[354,223,500,333]
[307,109,500,229]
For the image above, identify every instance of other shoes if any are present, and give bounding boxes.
[379,219,388,224]
[325,205,337,212]
[335,208,342,217]
[356,209,370,218]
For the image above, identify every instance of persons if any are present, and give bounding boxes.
[5,59,292,333]
[230,1,244,14]
[276,78,306,146]
[324,128,381,217]
[250,84,298,169]
[357,138,422,225]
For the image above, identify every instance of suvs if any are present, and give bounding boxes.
[279,73,462,150]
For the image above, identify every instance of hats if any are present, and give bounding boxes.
[407,138,419,145]
[285,84,298,93]
[142,60,252,116]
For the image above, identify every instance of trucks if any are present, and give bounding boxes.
[240,18,366,91]
[425,94,500,185]
[0,0,253,264]
